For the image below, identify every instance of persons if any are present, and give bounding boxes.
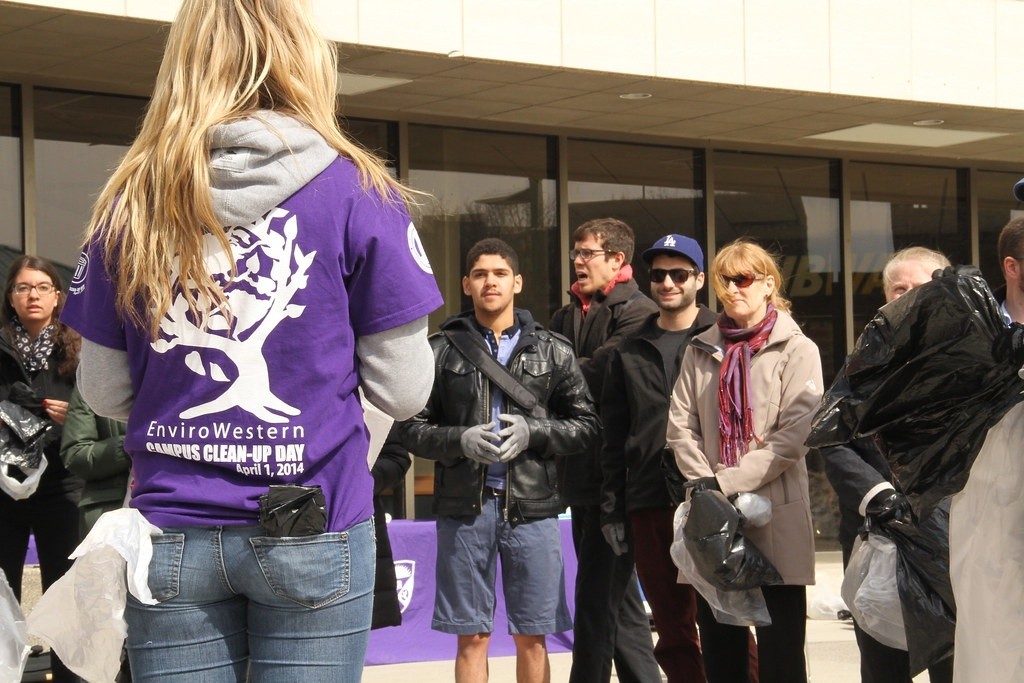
[0,255,132,683]
[550,217,722,683]
[383,237,604,683]
[371,423,411,631]
[666,239,824,683]
[61,0,444,683]
[803,179,1024,683]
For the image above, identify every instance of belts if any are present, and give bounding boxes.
[485,486,507,496]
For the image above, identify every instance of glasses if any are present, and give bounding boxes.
[13,282,54,295]
[569,248,616,260]
[722,275,754,287]
[649,269,694,283]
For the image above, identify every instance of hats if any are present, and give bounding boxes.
[641,234,703,272]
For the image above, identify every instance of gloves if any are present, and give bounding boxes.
[461,422,501,464]
[726,493,746,532]
[601,523,628,556]
[497,414,529,463]
[684,475,723,499]
